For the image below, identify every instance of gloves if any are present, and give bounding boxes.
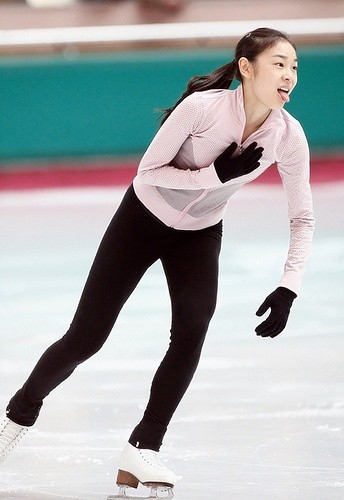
[255,287,297,339]
[214,142,264,184]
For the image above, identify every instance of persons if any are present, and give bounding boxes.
[0,27,315,500]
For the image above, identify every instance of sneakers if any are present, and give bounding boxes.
[108,441,174,499]
[1,415,31,463]
